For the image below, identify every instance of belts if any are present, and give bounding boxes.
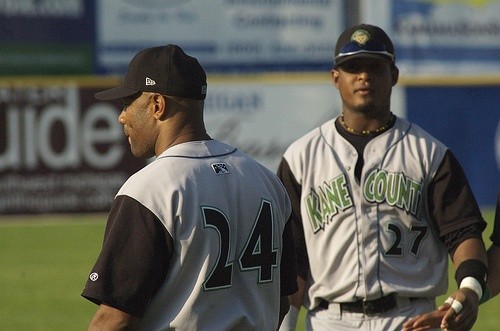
[319,295,417,313]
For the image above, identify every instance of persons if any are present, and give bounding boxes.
[80,44,297,331]
[276,24,489,331]
[402,196,500,331]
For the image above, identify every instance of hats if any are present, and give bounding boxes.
[93,43,208,101]
[332,22,396,67]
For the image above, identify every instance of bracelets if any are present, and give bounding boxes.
[454,258,489,300]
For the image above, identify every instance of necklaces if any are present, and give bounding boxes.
[340,109,393,134]
